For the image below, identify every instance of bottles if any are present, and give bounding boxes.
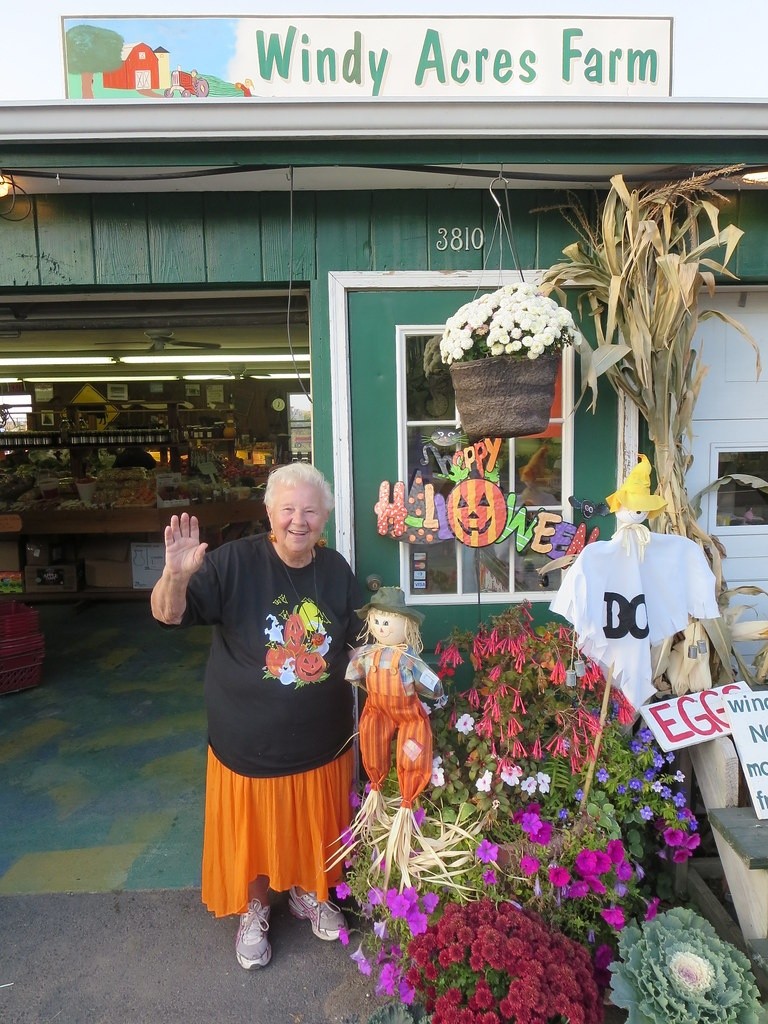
[0,430,60,445]
[72,429,168,444]
[183,424,221,438]
[59,414,73,445]
[308,452,311,463]
[298,452,302,461]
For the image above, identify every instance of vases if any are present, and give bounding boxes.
[450,354,560,442]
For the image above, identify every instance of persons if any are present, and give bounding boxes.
[744,506,753,525]
[111,445,156,469]
[0,447,32,467]
[151,463,374,971]
[519,447,560,506]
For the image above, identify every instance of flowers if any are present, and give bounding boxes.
[323,601,764,1024]
[439,281,583,365]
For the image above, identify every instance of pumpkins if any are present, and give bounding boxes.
[223,427,236,438]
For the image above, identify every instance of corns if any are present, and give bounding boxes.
[729,620,768,642]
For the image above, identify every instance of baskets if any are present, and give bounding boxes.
[0,597,46,695]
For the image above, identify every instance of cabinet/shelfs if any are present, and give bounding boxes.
[0,443,279,602]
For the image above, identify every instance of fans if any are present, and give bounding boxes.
[215,366,271,378]
[94,329,221,352]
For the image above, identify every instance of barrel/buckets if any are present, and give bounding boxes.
[40,478,60,498]
[74,478,96,500]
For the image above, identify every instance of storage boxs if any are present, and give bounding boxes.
[156,473,189,507]
[24,539,74,564]
[25,564,78,592]
[0,572,26,594]
[0,600,46,696]
[131,542,166,590]
[0,538,23,572]
[77,538,132,588]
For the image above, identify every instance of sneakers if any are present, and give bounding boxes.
[287,883,348,940]
[234,898,272,970]
